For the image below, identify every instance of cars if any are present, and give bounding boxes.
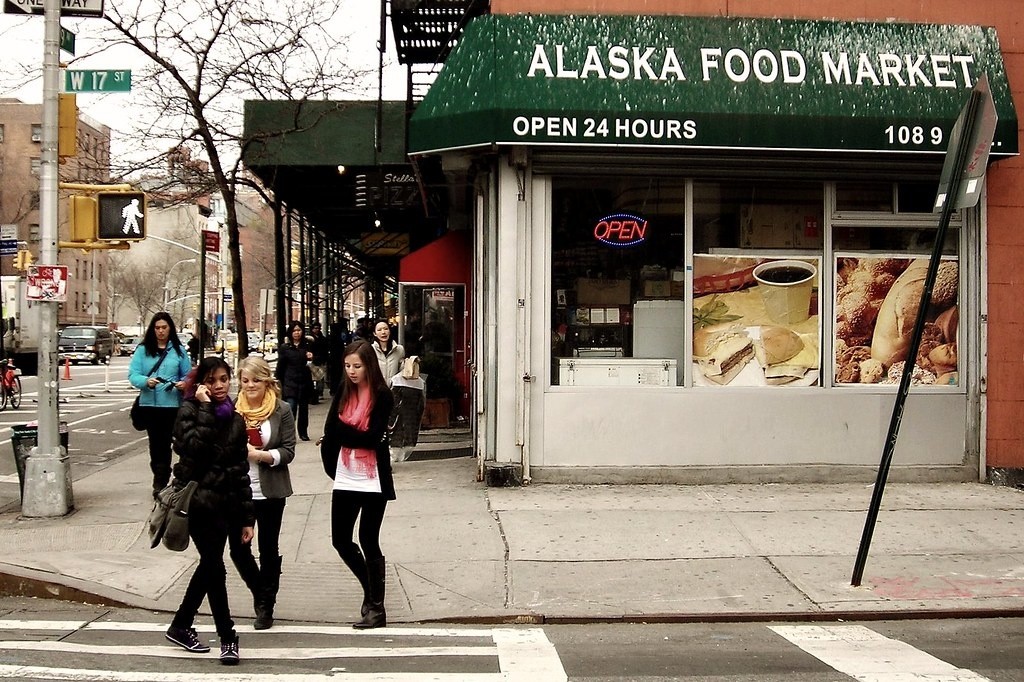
[176,333,194,352]
[216,330,278,354]
[119,338,141,355]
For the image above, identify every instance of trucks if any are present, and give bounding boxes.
[1,275,38,374]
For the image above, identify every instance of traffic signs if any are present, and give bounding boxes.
[64,69,131,92]
[3,0,103,19]
[59,26,76,57]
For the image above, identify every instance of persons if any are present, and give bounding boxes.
[0,338,18,405]
[186,319,425,473]
[165,356,297,666]
[128,311,193,504]
[316,340,401,631]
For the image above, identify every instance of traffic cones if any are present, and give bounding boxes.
[4,358,16,387]
[61,359,72,380]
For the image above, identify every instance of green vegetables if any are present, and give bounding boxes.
[693,294,744,331]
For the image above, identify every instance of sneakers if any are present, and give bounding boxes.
[164,626,210,653]
[219,637,239,666]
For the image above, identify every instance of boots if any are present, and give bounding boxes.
[339,543,370,618]
[353,556,387,630]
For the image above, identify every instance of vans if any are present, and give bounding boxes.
[58,326,113,365]
[182,317,196,334]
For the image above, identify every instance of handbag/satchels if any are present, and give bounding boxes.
[148,480,199,552]
[130,396,147,430]
[306,359,326,381]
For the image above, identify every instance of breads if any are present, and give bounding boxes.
[836,258,958,385]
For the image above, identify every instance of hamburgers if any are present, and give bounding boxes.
[693,322,819,385]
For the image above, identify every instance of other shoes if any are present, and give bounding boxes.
[253,601,274,630]
[318,395,324,401]
[300,434,310,441]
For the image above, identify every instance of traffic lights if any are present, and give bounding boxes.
[193,302,196,312]
[13,251,24,270]
[25,252,33,270]
[97,191,146,240]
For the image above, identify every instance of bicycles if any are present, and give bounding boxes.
[0,359,22,411]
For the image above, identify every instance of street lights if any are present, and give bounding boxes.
[164,258,195,310]
[112,294,120,356]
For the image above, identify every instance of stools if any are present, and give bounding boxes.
[421,398,450,428]
